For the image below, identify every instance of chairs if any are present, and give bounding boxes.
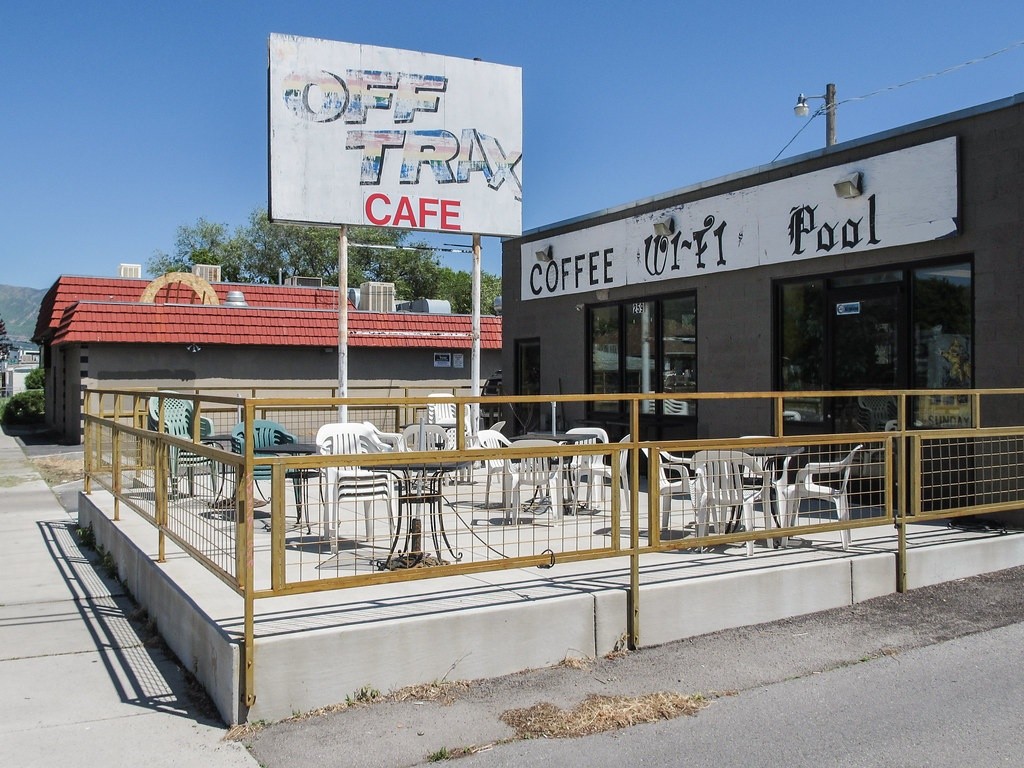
[146,393,864,571]
[648,390,689,416]
[853,388,923,463]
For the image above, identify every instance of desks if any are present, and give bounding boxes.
[360,461,464,571]
[509,434,599,515]
[724,447,804,547]
[252,443,325,535]
[200,433,271,509]
[399,423,458,449]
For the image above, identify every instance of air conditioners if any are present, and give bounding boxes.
[191,264,221,282]
[284,275,323,287]
[359,281,396,313]
[120,264,142,278]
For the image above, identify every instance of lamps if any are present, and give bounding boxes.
[186,344,201,353]
[794,94,826,117]
[652,215,675,237]
[534,244,554,262]
[834,172,863,199]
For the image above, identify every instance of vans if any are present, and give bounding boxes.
[479,371,504,429]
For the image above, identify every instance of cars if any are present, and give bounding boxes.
[815,335,970,423]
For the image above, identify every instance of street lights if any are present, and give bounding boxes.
[793,83,838,149]
